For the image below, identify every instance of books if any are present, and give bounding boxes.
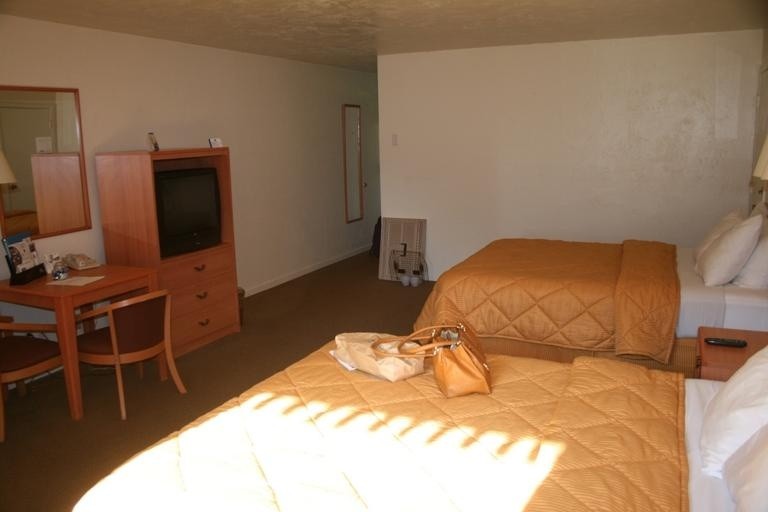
[2,232,40,275]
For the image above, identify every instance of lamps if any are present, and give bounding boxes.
[0,148,18,236]
[746,135,767,205]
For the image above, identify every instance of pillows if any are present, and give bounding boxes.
[722,424,767,511]
[697,346,768,477]
[691,209,742,272]
[694,216,766,289]
[731,202,768,291]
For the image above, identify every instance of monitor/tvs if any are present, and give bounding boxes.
[155,166,224,259]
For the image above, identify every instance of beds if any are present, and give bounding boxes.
[1,208,39,237]
[412,216,768,367]
[68,333,765,511]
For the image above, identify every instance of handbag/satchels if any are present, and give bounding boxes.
[369,216,380,257]
[432,320,491,398]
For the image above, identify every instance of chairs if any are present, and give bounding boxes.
[75,290,188,422]
[1,315,62,443]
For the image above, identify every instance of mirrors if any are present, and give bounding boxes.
[343,104,365,223]
[1,87,94,246]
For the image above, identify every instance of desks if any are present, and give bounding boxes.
[0,265,170,420]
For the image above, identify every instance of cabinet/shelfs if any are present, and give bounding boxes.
[32,153,87,234]
[93,147,242,360]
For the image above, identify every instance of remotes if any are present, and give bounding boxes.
[705,335,750,349]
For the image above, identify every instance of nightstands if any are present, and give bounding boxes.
[697,328,768,382]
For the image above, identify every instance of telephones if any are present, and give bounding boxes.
[62,253,100,270]
